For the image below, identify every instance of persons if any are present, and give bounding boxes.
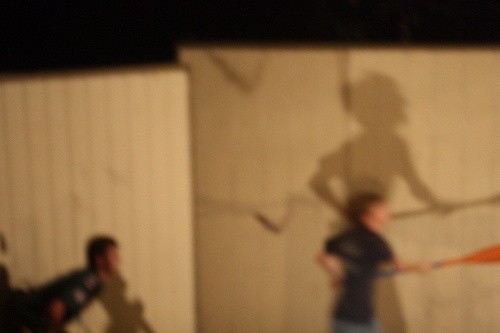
[315,192,432,333]
[12,236,121,333]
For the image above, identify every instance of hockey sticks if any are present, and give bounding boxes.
[333,242,500,281]
